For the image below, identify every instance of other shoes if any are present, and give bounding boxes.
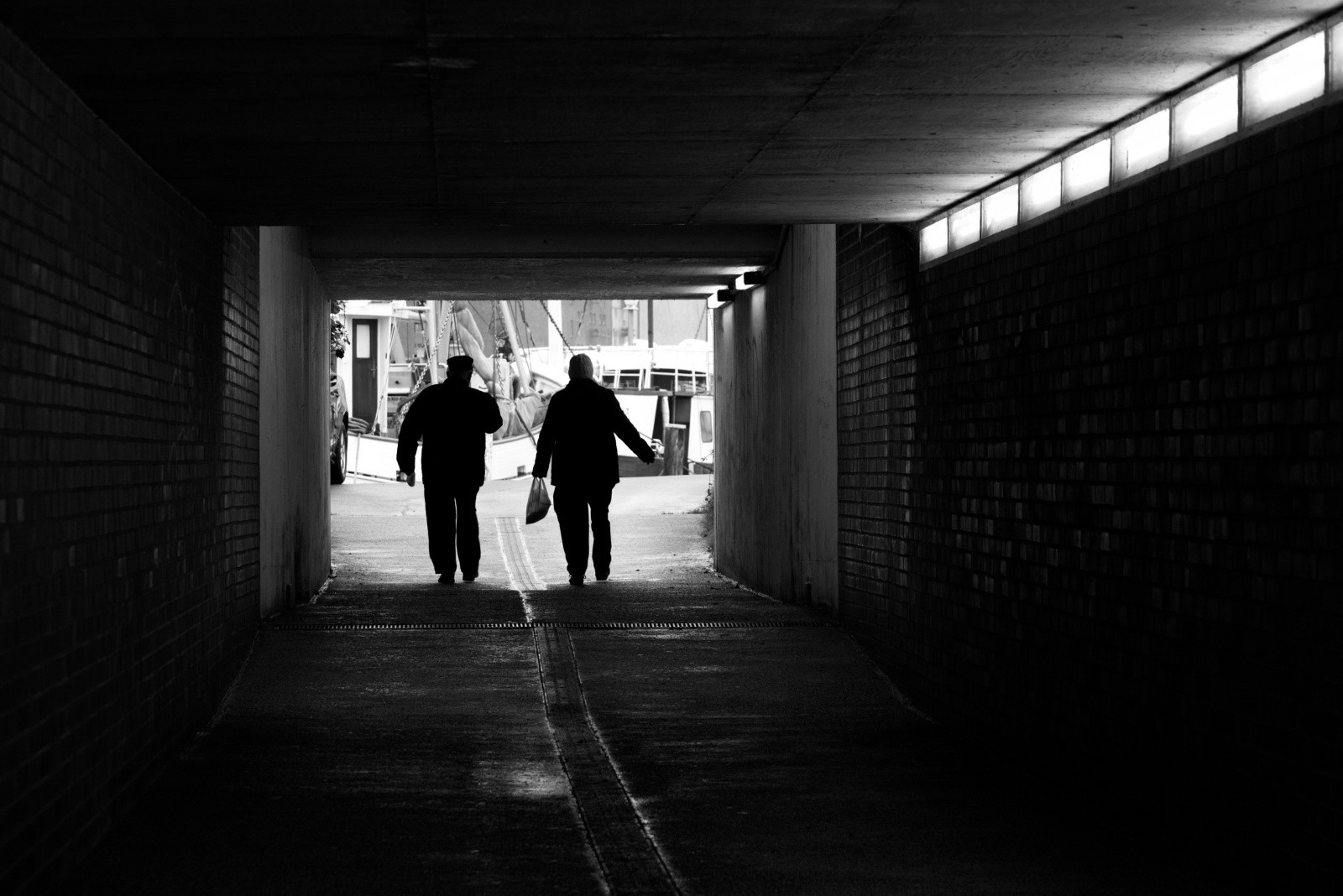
[570,574,583,586]
[438,572,454,586]
[596,567,610,581]
[463,571,475,581]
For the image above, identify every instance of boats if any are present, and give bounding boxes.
[333,300,713,483]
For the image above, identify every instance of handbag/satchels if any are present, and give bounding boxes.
[527,476,550,524]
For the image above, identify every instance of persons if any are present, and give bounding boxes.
[532,354,655,586]
[397,355,503,583]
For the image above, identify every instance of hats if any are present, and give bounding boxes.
[447,355,474,371]
[568,354,592,379]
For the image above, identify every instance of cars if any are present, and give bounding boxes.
[329,367,348,483]
